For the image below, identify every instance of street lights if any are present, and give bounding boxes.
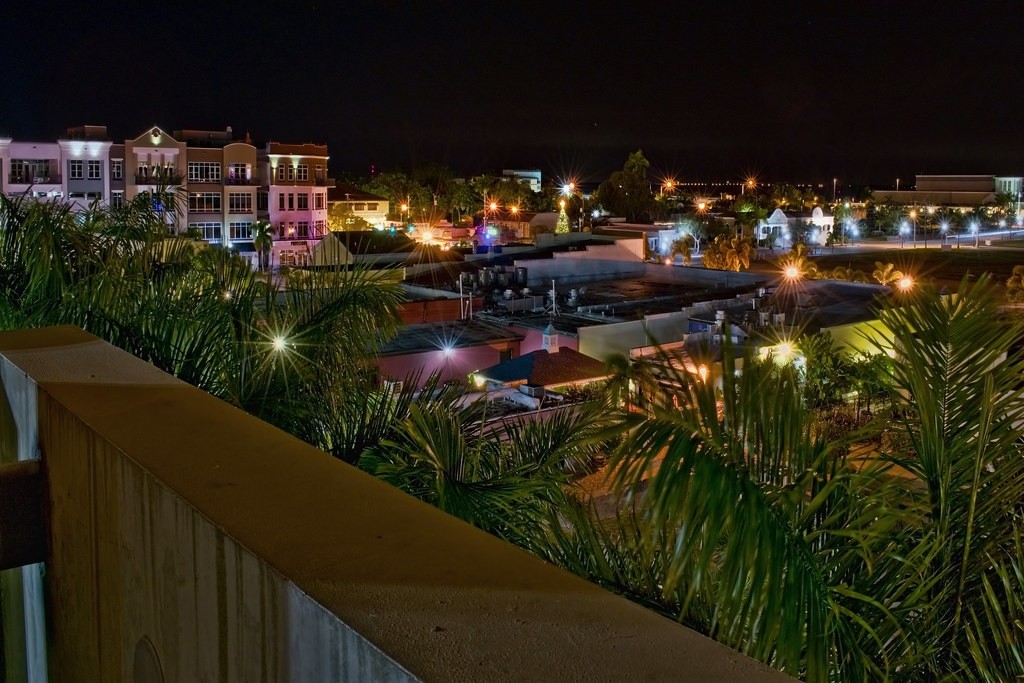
[511,204,521,235]
[909,210,916,248]
[844,202,859,245]
[999,219,1007,240]
[489,202,497,227]
[900,221,911,248]
[562,182,575,229]
[970,223,978,246]
[928,207,935,240]
[941,222,947,246]
